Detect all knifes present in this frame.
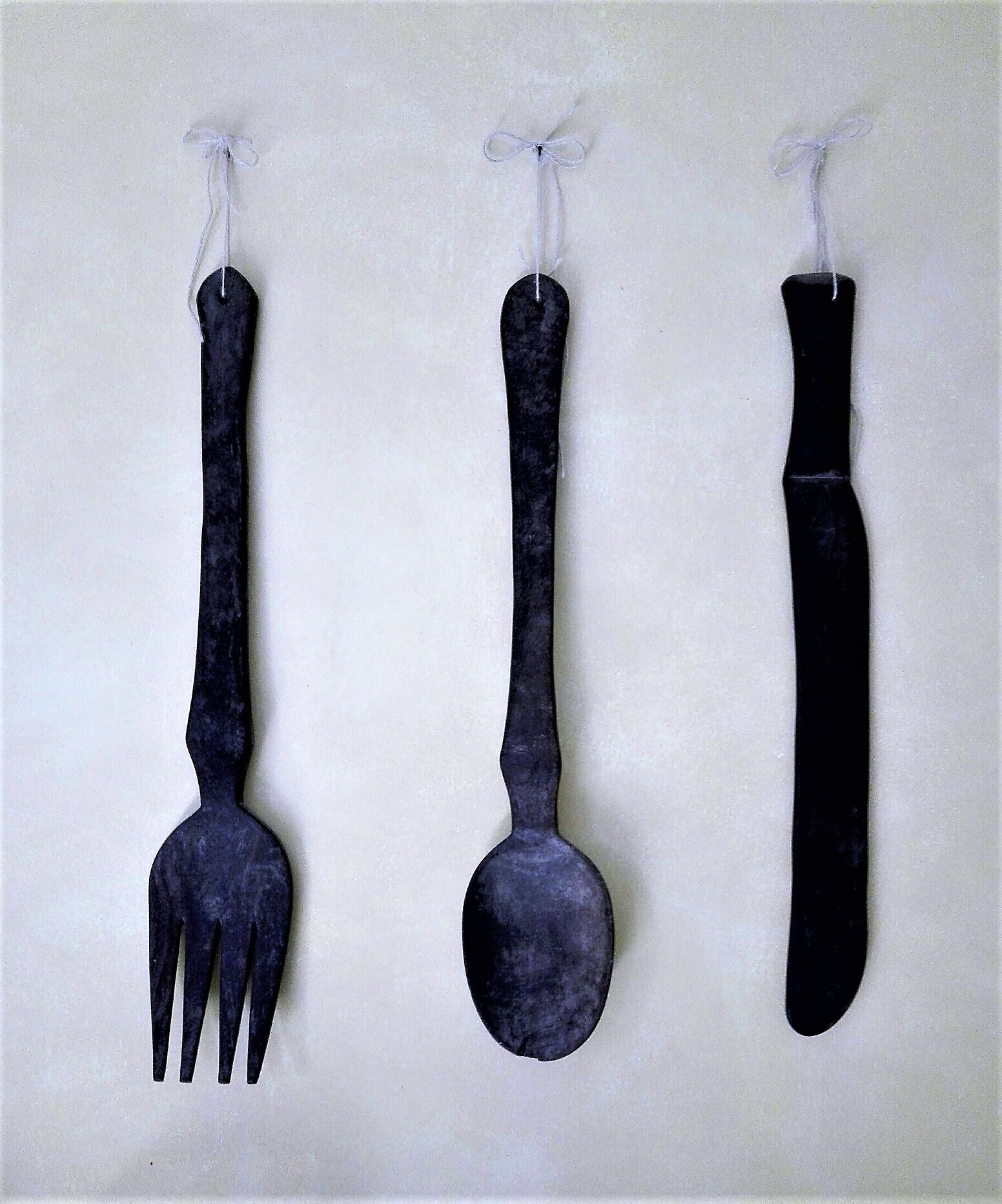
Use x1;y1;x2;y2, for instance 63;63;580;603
780;273;869;1037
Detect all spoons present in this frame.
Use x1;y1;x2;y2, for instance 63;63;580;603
461;271;616;1062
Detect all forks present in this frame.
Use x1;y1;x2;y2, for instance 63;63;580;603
148;265;296;1083
